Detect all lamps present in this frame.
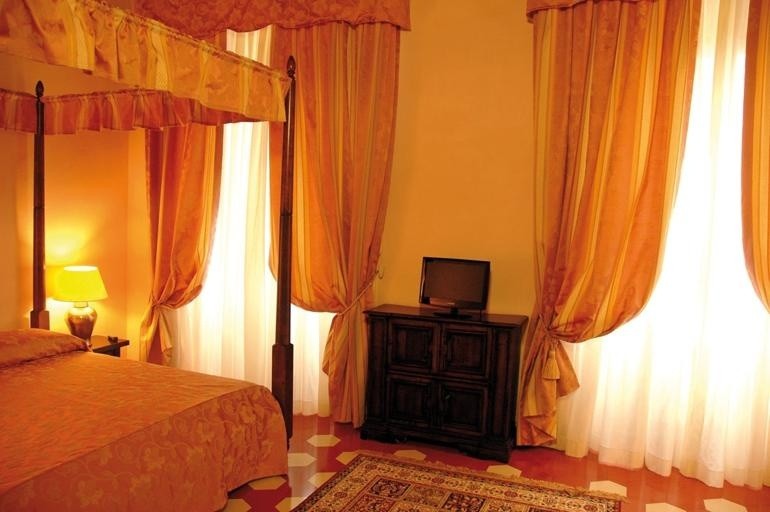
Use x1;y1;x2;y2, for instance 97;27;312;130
51;264;109;337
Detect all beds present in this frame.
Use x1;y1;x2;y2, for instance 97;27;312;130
0;311;295;512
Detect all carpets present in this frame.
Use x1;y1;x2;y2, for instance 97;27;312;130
288;451;623;512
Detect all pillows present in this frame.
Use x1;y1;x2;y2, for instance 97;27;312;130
0;327;91;369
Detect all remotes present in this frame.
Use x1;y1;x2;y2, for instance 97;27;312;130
108;336;118;342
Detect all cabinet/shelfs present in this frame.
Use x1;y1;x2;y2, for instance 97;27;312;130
361;304;529;464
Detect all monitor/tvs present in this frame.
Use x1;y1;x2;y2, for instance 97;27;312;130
419;257;490;319
